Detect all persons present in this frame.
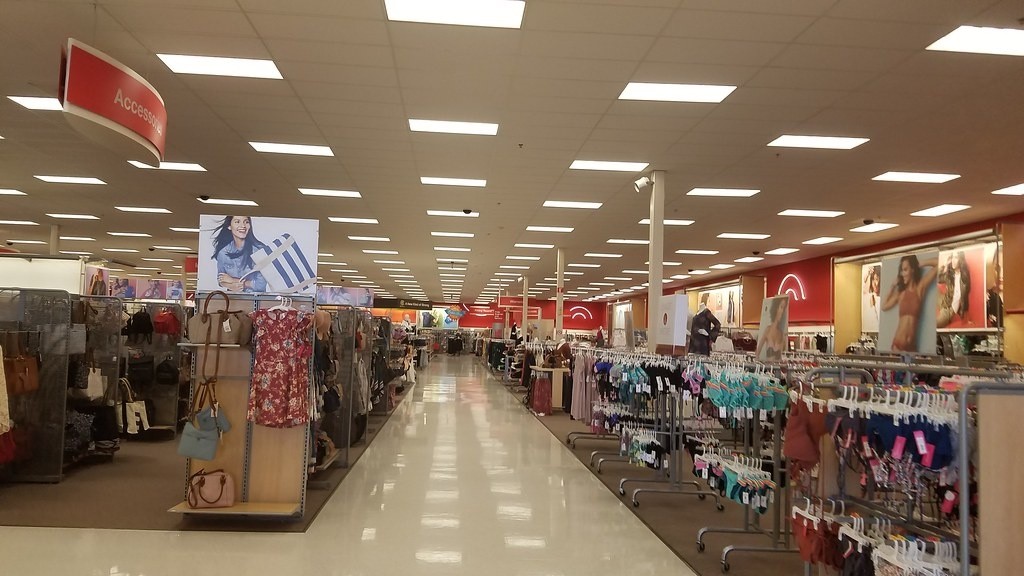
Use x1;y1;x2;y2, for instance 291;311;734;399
596;326;606;344
87;268;106;295
211;217;271;293
688;302;721;354
166;280;183;300
756;299;788;363
863;266;882;326
317;286;374;306
510;322;519;340
141;280;163;299
555;338;573;367
402;313;413;331
883;256;937;351
114;279;134;298
938;253;977;327
987;245;1006;327
726;291;738;326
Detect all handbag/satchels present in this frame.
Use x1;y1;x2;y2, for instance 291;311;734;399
67;348;107;401
38;300;86;356
116;378;150;434
4;330;39;396
187;468;234;509
196;378;232;437
188;291;252;345
83;382;118;442
177;382;218;460
238;232;318;294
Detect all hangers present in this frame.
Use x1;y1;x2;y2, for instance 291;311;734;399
570;346;676;374
592;399;661;447
683;352;1024;433
686;430;771;484
280;297;293;311
266;295;288;312
524;341;557;378
792;497;961;576
286;298;296;311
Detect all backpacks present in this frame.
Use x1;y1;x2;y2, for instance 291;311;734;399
157;357;179;385
132;306;154;345
154;306;181;339
121;305;132;341
128;351;155;386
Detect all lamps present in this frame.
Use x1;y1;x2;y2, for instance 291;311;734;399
633;176;654;193
